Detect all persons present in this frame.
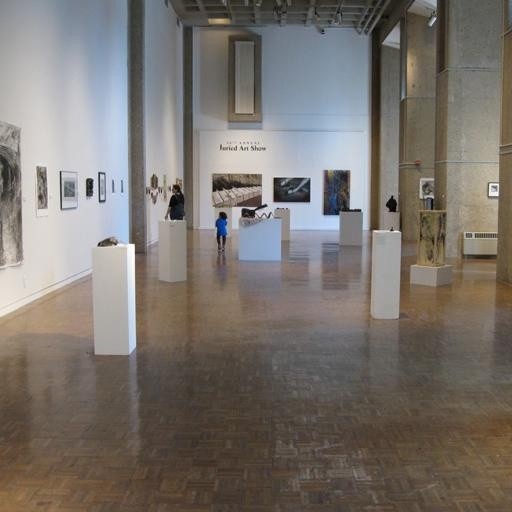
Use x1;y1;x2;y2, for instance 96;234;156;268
164;184;185;220
214;212;228;252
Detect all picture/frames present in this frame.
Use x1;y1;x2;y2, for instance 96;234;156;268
60;170;79;210
419;177;434;200
98;171;107;202
487;182;500;197
